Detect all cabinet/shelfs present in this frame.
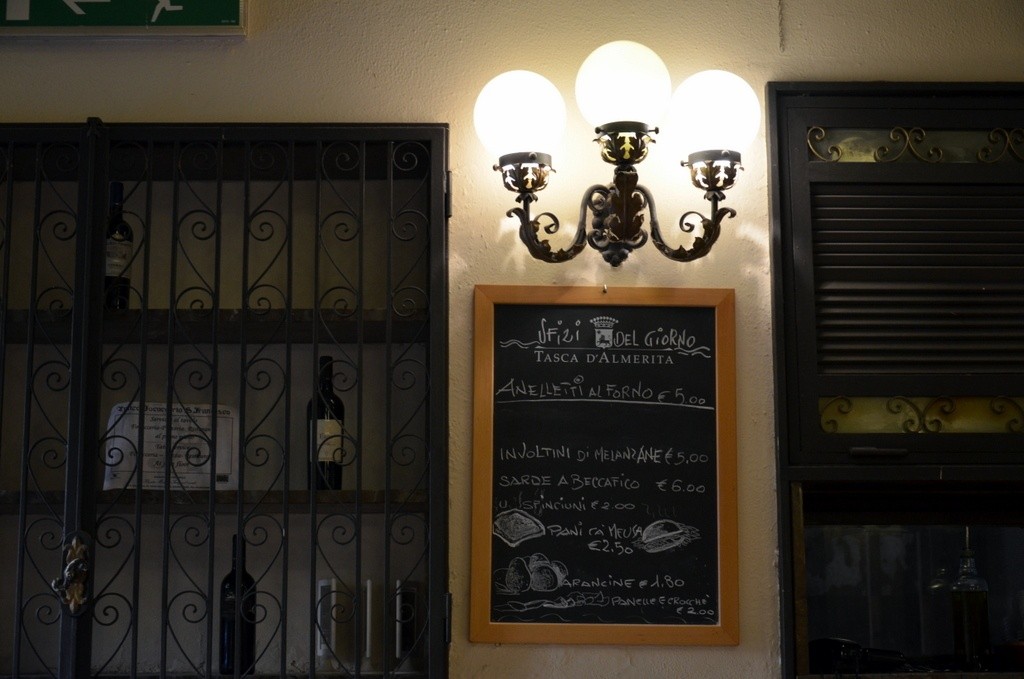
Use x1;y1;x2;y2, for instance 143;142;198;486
2;121;445;679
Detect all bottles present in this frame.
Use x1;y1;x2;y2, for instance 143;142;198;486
306;355;344;491
103;181;134;311
219;533;257;676
951;526;994;672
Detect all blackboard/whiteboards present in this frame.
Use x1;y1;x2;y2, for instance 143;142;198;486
469;284;741;647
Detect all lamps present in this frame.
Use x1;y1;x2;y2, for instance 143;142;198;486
472;40;760;268
